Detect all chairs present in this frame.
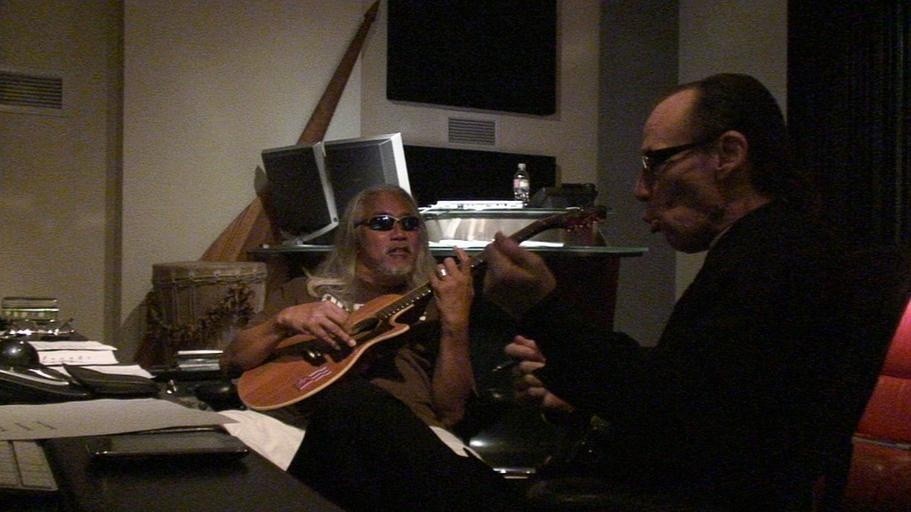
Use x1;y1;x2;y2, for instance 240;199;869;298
812;259;911;511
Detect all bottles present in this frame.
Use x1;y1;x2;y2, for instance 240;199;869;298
514;163;529;207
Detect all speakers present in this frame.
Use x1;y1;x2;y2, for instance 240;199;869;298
260;140;339;245
323;131;412;222
403;144;557;207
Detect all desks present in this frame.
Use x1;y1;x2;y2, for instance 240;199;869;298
0;396;345;512
246;244;649;330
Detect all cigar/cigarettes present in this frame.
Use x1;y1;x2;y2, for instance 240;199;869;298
492;356;520;374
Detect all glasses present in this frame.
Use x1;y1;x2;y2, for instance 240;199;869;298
640;137;708;171
353;213;420;233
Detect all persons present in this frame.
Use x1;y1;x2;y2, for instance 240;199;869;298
216;183;479;512
481;72;892;511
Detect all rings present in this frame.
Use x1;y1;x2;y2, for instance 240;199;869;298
439;269;450;278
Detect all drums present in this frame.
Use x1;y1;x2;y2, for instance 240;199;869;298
153;262;267;366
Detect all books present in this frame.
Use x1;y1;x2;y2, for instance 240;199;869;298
435;198;524;211
1;295;224;396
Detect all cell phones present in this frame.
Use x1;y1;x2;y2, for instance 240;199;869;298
82;432;253;465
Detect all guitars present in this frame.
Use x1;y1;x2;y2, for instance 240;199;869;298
238;206;612;424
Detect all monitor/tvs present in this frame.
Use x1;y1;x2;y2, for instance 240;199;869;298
386;0;558;116
